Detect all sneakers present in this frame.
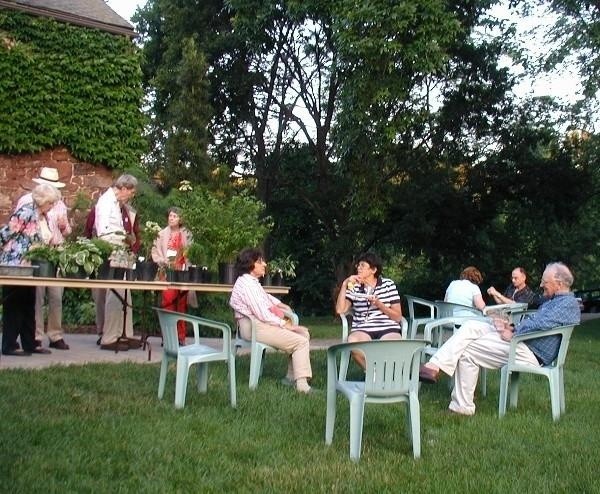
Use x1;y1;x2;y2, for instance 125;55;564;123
3;331;143;356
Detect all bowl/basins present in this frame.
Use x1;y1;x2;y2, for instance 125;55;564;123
0;264;40;277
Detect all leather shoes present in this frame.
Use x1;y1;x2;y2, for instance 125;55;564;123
417;365;440;382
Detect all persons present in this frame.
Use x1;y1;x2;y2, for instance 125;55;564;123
419;260;582;416
336;251;403;371
149;206;196;347
0;181;62;356
93;172;141;351
486;266;536;327
229;246;323;394
14;167;72;349
85;179;140;345
443;265;487;330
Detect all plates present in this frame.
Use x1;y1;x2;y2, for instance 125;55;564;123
350;292;375;298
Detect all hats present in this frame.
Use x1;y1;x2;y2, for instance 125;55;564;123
31;168;66;188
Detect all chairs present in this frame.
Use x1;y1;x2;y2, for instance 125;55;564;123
151;307;237;409
233;306;300;390
404;294;440;342
422;317;493;398
487;303;529;328
337;310;409;385
324;338;431;460
497;322;582;422
435;299;484;347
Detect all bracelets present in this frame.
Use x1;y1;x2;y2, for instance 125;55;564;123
497;293;503;298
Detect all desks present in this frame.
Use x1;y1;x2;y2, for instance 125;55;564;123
1;275;292;360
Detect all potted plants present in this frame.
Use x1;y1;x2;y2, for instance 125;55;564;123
27;179;298;286
